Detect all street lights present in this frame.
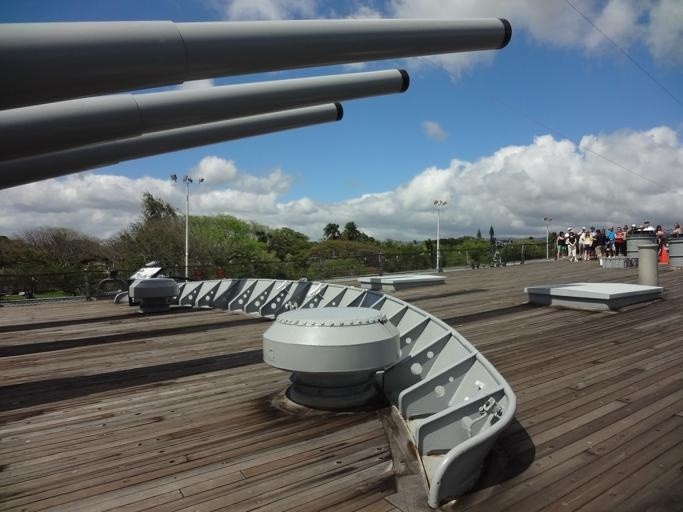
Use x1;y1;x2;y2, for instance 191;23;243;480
544;217;553;260
434;199;448;268
170;174;204;283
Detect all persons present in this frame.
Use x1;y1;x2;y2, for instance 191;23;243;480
556;222;683;266
